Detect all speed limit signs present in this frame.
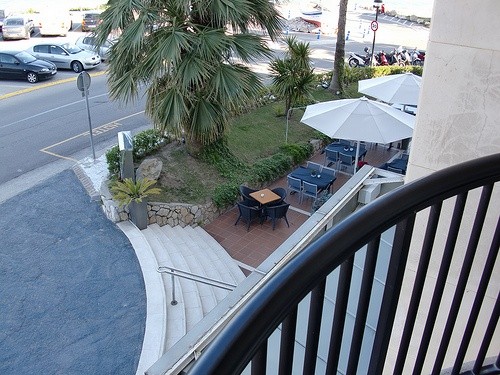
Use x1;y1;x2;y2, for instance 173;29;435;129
371;20;378;31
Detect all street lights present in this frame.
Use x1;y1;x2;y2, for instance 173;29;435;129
370;0;382;69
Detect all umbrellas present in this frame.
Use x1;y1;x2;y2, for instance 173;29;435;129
299;96;415;173
359;72;422;112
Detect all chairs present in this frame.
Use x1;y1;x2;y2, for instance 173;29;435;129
400;154;409;162
388;167;402;174
234;139;368;232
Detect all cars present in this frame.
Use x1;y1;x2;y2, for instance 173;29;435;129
38;10;74;36
0;50;58;84
1;15;35;40
23;41;102;73
0;9;8;31
81;12;103;32
74;33;119;61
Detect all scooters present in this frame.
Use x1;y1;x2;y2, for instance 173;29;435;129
347;47;426;68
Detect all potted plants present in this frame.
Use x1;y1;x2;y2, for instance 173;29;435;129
112;176;163;231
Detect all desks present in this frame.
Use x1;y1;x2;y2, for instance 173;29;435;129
326;143;368;170
292;168;336;202
390;159;407;171
249;188;282;225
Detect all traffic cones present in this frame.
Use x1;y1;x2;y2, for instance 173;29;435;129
346;31;351;40
316;30;321;39
285;27;289;35
